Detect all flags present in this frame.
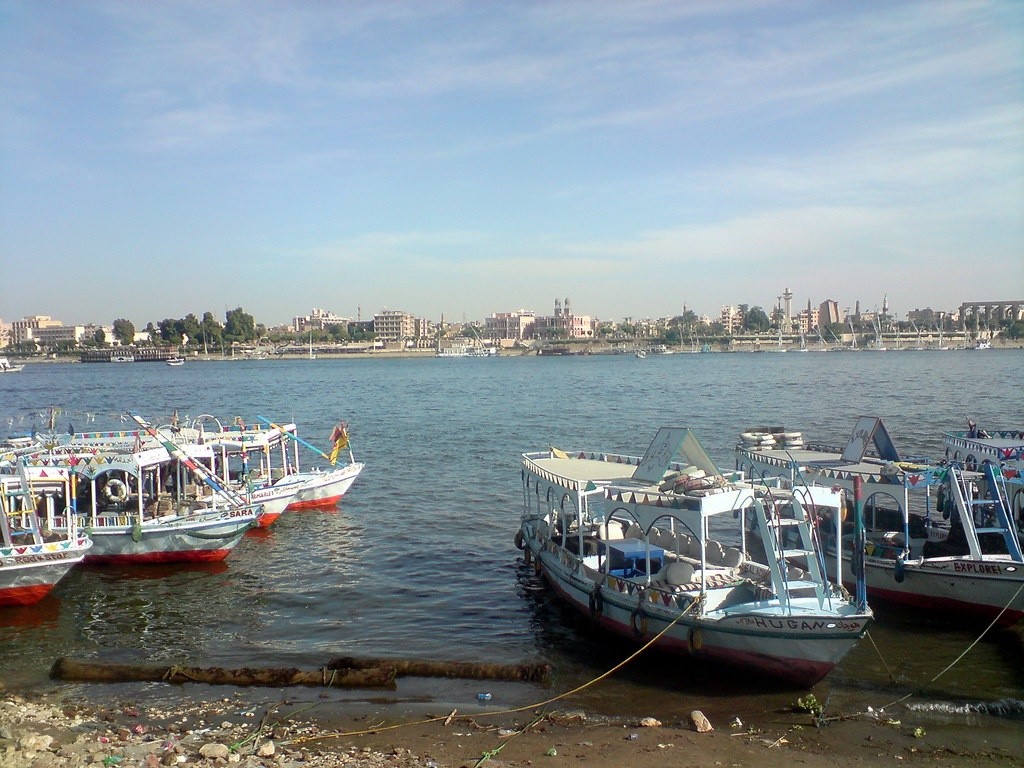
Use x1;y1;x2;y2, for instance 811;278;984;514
329;423;350;466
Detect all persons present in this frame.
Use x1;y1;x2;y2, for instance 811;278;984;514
763;499;855;537
38;456;220;519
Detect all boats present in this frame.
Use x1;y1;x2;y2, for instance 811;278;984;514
942;415;1024;544
111;355;136;363
736;414;1024;625
0;355;26;373
165;358;184;365
634;350;646;359
0;410;365;609
434;345;490;357
512;428;874;690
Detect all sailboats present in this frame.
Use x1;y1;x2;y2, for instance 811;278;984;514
767;317;991;352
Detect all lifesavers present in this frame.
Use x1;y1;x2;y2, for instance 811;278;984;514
741;430;805;452
683;625;701;656
105;479;127;503
533;555;543;579
523;544;532;566
629;606;648;637
659;465;736;498
514;532;527;551
587;589;603;620
